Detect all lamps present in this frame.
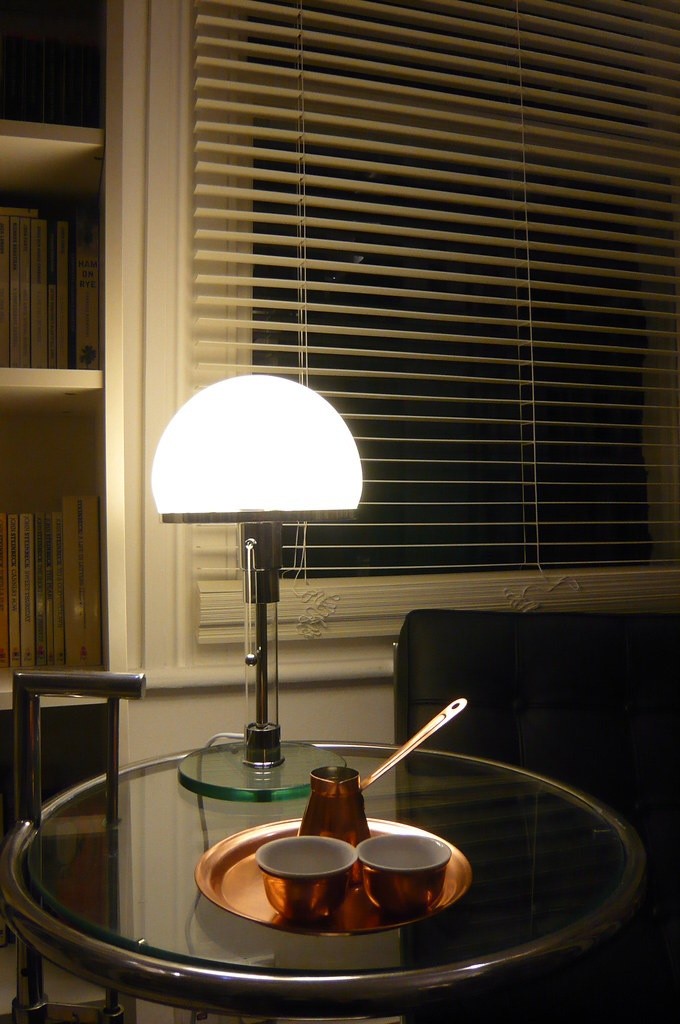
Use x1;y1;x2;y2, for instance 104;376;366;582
151;375;364;800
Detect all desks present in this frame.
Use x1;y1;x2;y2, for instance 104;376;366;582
0;741;647;1024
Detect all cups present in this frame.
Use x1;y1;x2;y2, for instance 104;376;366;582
358;835;452;913
258;837;357;924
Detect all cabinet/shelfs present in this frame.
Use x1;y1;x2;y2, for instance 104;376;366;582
0;0;124;953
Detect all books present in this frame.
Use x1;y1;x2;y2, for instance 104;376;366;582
0;206;105;370
0;495;101;668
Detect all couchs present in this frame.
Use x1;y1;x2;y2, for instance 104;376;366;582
395;608;680;1024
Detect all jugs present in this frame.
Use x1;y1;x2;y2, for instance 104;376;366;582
297;698;467;888
